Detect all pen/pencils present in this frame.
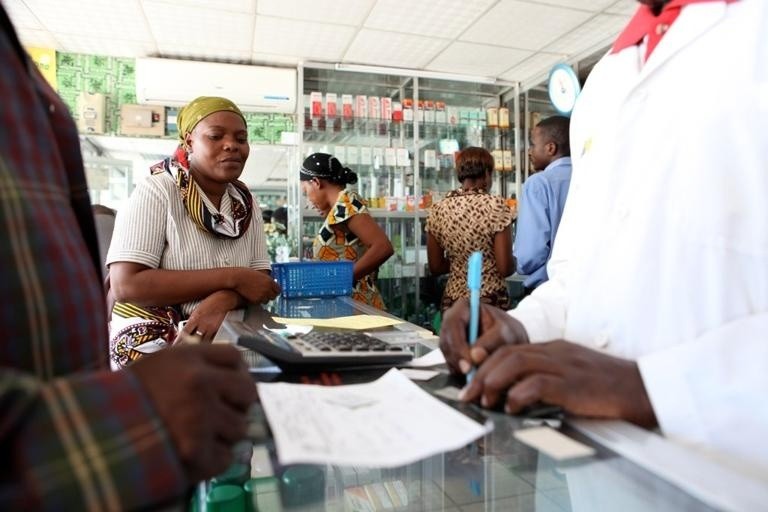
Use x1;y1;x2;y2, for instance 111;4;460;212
467;251;483;386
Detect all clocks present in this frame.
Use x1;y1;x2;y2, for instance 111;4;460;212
545;63;579;119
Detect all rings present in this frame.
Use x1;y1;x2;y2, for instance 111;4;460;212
194;328;205;338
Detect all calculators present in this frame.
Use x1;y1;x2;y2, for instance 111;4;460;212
223;322;415;368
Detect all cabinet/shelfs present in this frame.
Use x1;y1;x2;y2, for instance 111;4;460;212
301;69;513;333
157;292;767;512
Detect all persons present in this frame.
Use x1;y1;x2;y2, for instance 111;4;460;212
298;152;396;313
436;1;768;435
426;143;517;325
105;93;283;374
512;114;575;298
0;3;263;512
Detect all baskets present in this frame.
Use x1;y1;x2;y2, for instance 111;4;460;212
270;261;352;298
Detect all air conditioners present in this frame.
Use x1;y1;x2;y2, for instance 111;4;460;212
133;55;296;114
